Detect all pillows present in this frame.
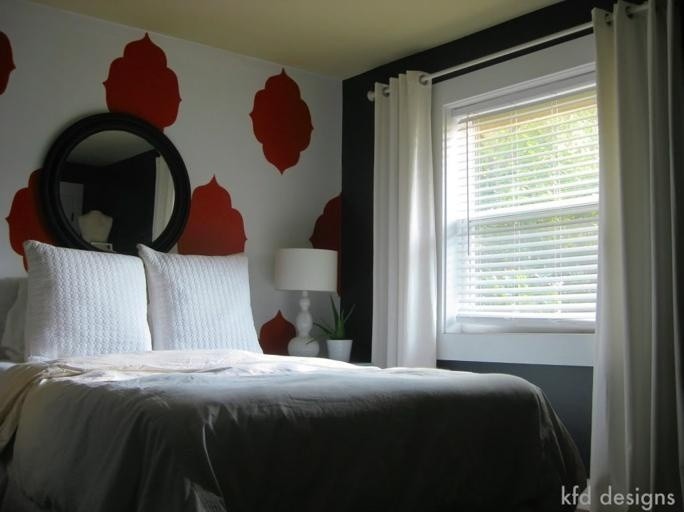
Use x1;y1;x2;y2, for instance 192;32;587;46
22;239;263;362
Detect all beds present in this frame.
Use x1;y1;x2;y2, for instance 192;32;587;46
0;352;549;512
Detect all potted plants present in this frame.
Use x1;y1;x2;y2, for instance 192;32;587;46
305;294;356;363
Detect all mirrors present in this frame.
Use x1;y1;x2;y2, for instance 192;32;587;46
40;112;190;258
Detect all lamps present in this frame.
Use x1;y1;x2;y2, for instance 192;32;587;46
273;247;339;356
